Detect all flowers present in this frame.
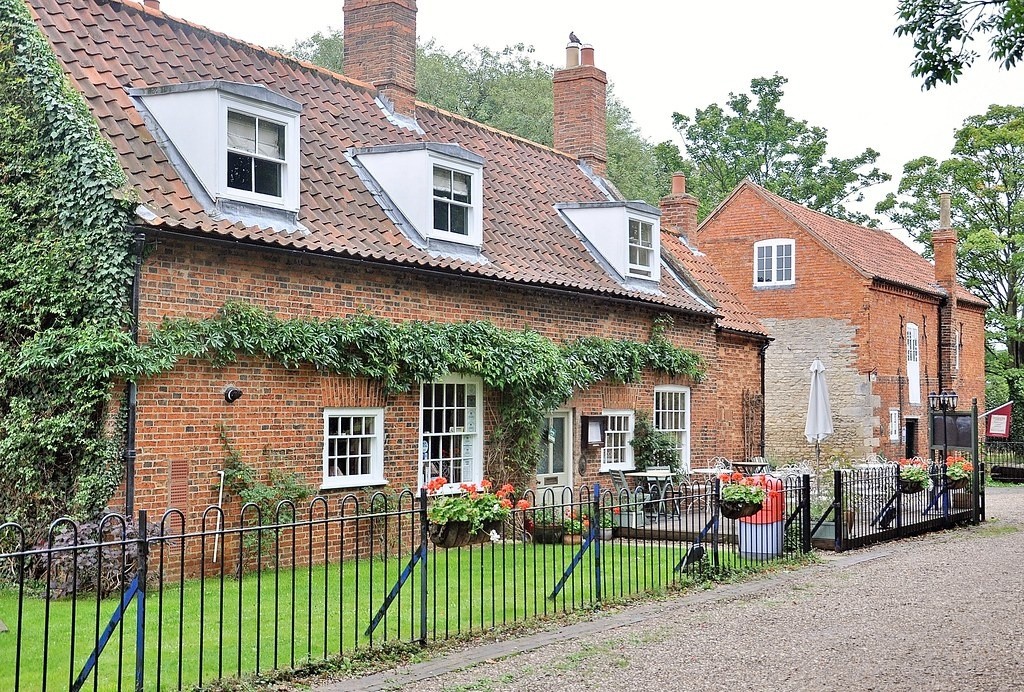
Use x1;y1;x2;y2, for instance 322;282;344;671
945;456;974;481
898;458;931;489
424;477;529;544
720;473;779;505
530;502;621;535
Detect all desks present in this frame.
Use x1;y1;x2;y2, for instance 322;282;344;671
695;469;733;473
625;472;677;518
732;461;769;477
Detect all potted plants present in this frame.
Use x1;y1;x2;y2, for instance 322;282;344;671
609;504;644;529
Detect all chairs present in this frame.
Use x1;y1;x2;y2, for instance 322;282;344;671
680;456;932;515
609;465;681;524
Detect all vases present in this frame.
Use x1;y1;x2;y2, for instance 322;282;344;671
428;520;504;548
947;477;968;489
535;525;562;543
900;478;930;494
719;499;763;518
600;528;613;540
563;534;583;545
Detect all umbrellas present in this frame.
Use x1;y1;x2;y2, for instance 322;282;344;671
804;356;835;500
